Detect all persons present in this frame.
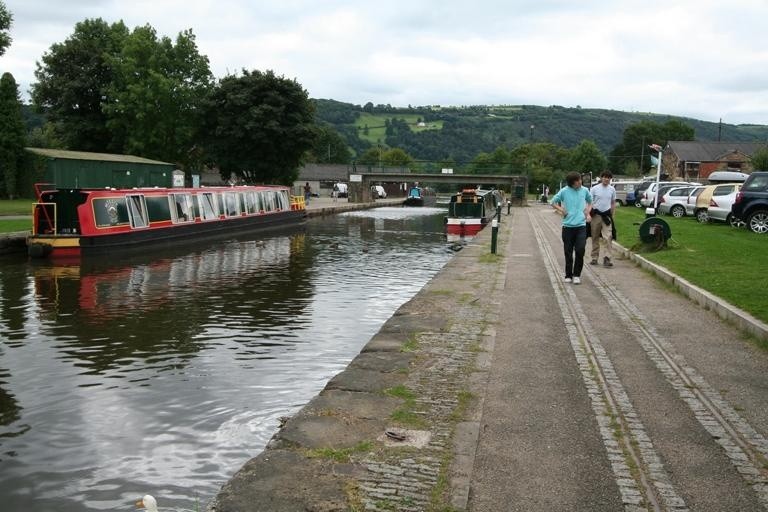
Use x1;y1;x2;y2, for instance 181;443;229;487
589;169;617;266
304;181;310;205
332;185;339;202
548;171;593;283
535;184;540;200
544;186;548;198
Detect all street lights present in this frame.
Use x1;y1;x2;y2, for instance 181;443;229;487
646;141;663;215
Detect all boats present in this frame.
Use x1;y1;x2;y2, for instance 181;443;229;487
29;228;312;320
402;186;438;207
26;183;311;252
444;224;482;254
443;186;507;227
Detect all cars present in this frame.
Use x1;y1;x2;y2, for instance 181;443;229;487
333;183;348;197
370;185;387;199
310;190;321;198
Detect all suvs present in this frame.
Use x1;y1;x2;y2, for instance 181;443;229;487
595;168;749;235
730;171;767;234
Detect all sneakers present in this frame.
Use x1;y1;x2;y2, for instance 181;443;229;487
603;257;613;266
573;277;580;284
590;260;598;265
565;278;572;282
